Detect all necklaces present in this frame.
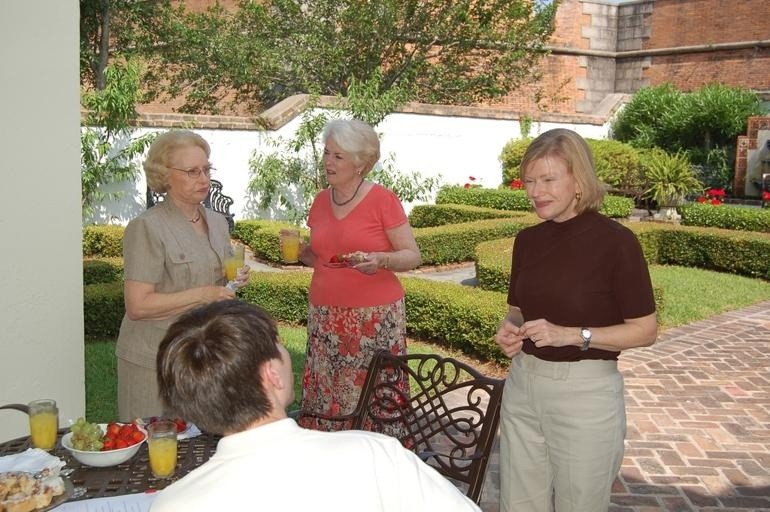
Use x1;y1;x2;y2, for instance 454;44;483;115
329;174;367;207
181;208;206;226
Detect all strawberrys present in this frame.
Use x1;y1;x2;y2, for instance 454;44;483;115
101;424;142;451
328;253;363;267
147;415;183;436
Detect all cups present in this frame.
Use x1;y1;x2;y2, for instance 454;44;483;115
222;245;245;283
27;399;60;451
147;420;177;477
280;229;300;263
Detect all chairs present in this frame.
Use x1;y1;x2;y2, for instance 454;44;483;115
292;347;505;508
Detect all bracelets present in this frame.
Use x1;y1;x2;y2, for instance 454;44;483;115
382;252;390;272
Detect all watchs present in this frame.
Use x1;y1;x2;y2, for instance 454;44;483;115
578;324;594;355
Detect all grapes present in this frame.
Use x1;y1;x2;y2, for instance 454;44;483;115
73;417;103;453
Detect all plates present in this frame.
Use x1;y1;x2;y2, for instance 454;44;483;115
146;415;192;434
0;473;76;512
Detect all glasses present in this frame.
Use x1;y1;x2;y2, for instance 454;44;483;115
166;166;218;179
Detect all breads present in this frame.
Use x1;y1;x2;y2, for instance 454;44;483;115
0;472;64;512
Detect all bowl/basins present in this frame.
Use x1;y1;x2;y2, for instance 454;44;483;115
59;422;149;468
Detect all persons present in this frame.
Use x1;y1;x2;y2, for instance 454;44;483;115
291;112;425;456
138;297;490;512
496;126;663;511
108;125;256;430
